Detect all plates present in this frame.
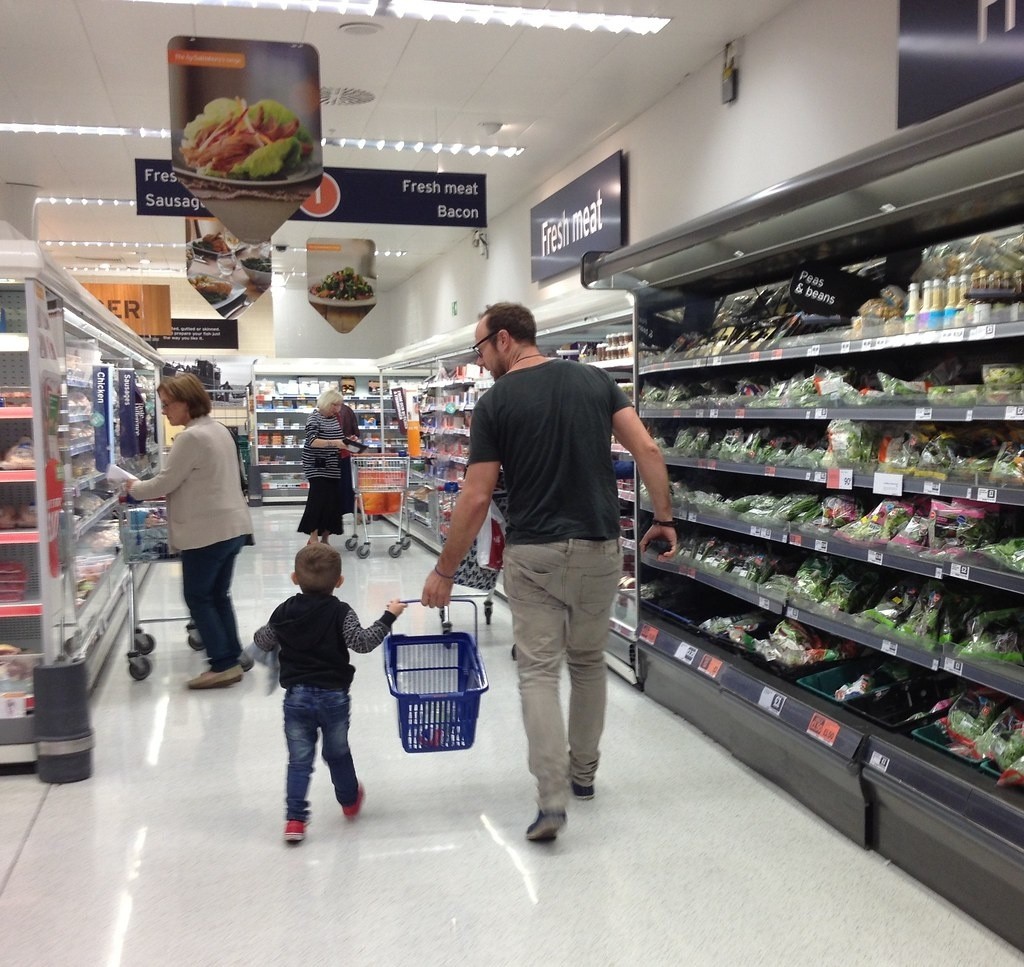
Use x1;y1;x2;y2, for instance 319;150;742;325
307;290;377;307
186;273;247;316
187;238;247;256
171;128;322;186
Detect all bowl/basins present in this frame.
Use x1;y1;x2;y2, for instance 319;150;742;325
241;263;272;284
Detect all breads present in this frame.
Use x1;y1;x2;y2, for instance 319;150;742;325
0;437;37;527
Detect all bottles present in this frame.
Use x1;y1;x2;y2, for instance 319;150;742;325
904;270;1024;334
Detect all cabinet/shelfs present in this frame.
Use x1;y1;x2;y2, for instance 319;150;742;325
0;81;1024;967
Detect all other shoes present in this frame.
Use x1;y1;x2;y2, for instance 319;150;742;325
201;654;255;676
342;782;365;819
570;781;595;800
525;810;566;840
284;821;306;841
188;664;243;688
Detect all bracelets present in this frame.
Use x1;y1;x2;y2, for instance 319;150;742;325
651;518;678;527
435;566;455;578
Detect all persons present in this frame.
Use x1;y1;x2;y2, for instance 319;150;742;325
254;543;408;841
422;303;677;841
297;390;358;546
126;374;256;689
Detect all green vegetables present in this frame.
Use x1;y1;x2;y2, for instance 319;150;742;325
317;267;374;300
181;96;310;180
244;257;271;273
643;367;1024;786
192;241;213;248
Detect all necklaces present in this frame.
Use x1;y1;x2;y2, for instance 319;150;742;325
516;355;545;362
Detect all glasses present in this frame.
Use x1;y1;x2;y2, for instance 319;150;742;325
472;329;500;358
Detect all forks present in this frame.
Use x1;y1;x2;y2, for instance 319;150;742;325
223;294;255;318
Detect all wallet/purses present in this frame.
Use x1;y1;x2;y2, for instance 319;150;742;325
314;457;326;468
